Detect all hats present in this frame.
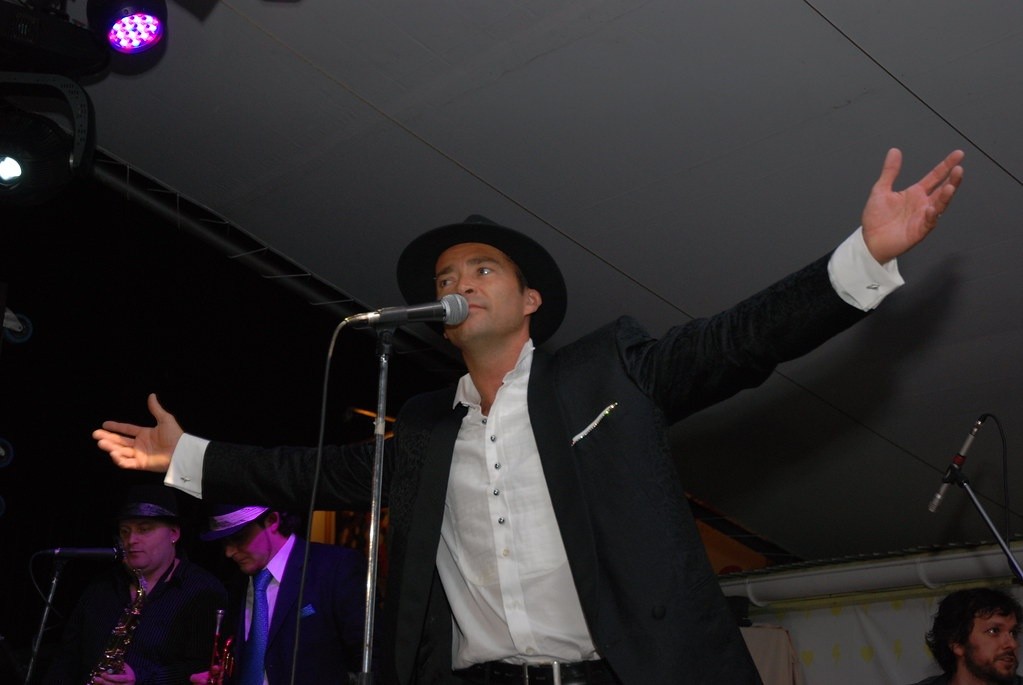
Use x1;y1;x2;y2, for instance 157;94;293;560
396;214;568;346
200;491;271;541
112;486;181;523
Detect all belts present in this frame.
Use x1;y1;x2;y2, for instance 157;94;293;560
452;658;617;685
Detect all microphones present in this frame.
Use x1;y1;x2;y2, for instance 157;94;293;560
41;548;118;559
345;293;469;326
928;413;989;513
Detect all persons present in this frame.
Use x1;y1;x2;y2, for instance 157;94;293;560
93;147;965;685
42;487;384;685
903;581;1023;685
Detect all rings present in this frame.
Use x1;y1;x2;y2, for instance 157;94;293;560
937;212;943;220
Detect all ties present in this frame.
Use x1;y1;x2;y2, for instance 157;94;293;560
239;568;273;685
